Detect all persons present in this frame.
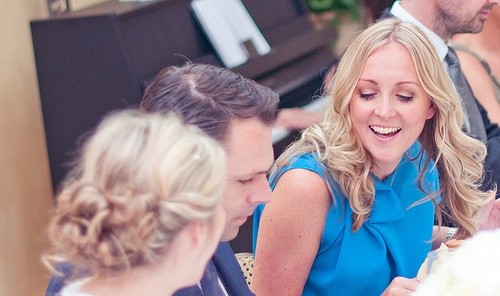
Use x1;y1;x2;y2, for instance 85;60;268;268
251;18;500;296
381;0;500;227
41;108;228;296
45;64;280;296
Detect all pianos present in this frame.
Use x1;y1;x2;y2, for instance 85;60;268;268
28;0;339;254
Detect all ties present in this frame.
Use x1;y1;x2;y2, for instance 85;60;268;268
200;257;226;296
444;51;488;145
